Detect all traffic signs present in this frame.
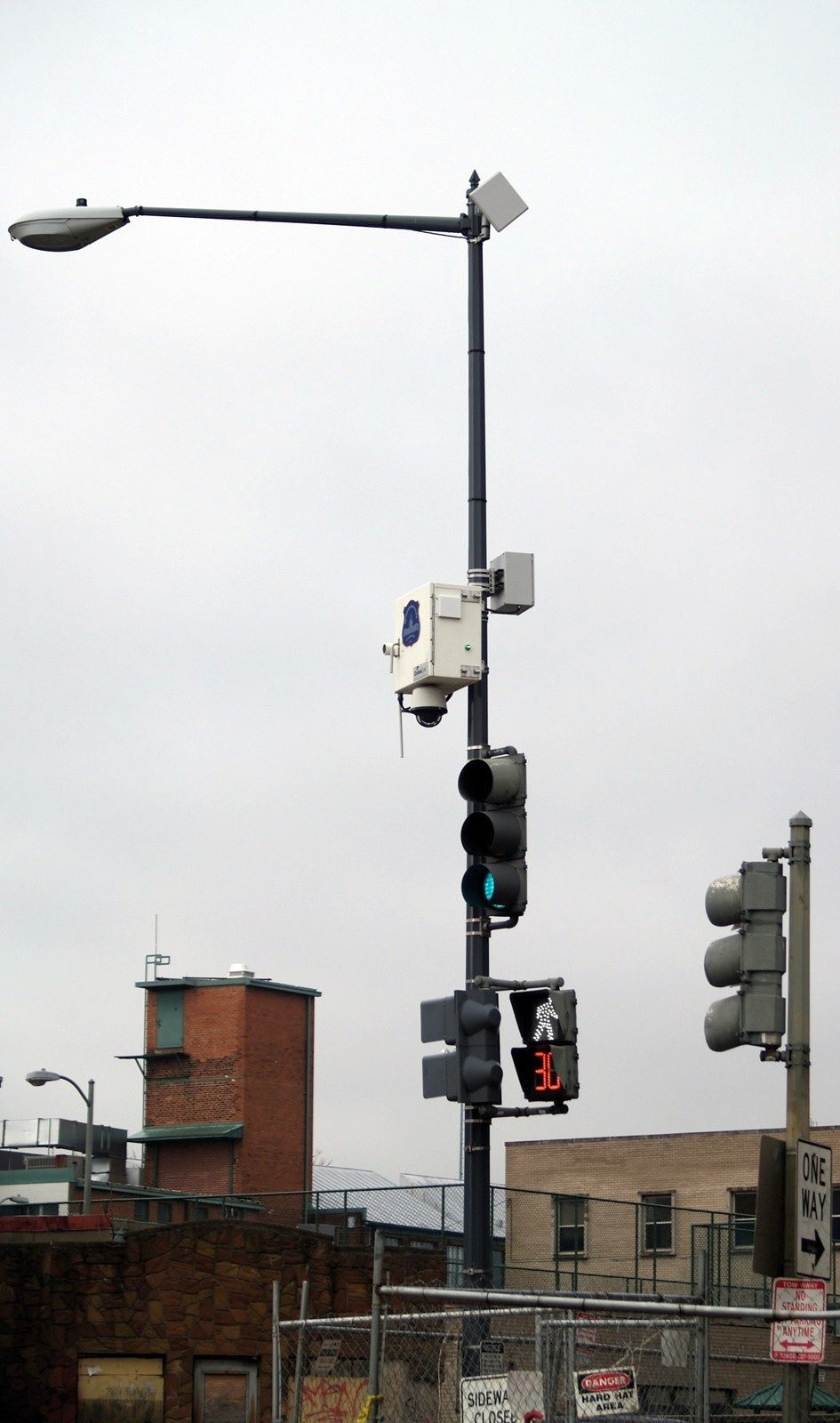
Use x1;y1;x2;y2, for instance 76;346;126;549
794;1136;834;1282
768;1277;827;1364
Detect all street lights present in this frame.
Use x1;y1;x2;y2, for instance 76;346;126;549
7;166;541;1377
25;1070;95;1215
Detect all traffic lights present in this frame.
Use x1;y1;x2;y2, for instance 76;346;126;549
456;751;528;917
419;987;501;1109
507;989;579;1102
702;860;788;1054
